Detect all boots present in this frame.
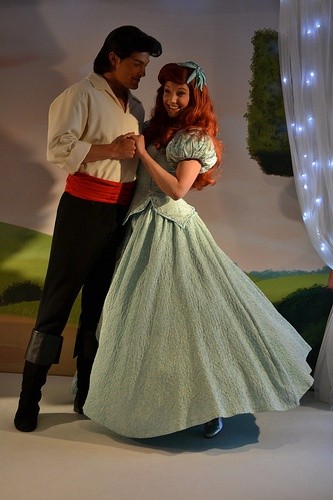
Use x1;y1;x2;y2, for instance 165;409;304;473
74;333;98;413
14;329;64;430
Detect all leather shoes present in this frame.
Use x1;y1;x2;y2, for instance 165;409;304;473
205;417;223;439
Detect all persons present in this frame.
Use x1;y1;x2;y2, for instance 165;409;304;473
81;60;315;440
12;24;162;432
